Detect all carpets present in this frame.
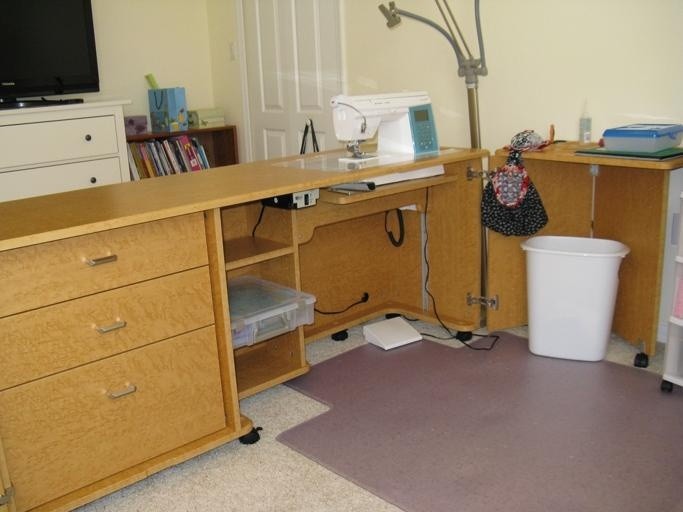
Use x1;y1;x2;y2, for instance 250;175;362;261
274;328;682;512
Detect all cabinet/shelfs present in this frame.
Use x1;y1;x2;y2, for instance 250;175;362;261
660;191;683;388
125;124;239;175
0;99;134;204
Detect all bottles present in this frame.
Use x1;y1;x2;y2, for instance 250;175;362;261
578;101;592;147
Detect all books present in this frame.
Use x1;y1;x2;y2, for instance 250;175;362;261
125;135;211;182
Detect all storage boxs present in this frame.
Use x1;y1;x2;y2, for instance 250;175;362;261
600;124;683;154
226;274;317;350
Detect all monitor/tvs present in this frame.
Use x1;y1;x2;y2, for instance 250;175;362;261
0;0;99;110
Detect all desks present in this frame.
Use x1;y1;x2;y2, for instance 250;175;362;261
0;144;490;512
483;140;683;367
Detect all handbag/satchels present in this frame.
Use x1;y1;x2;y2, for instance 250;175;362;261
481;158;547;236
148;88;188;133
